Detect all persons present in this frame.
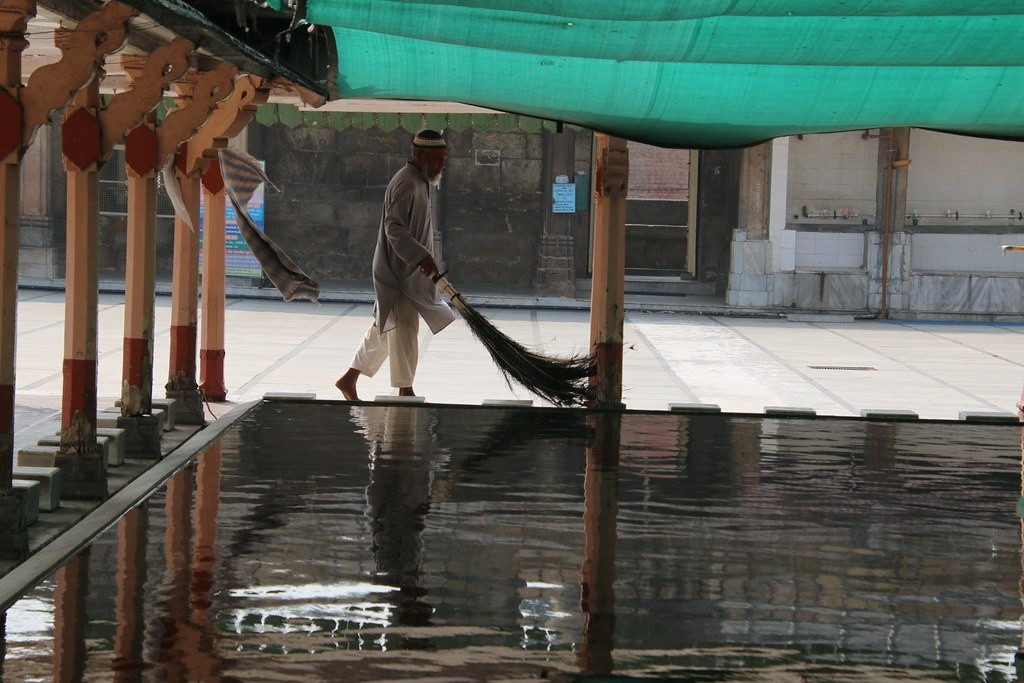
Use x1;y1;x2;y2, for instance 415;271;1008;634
335;130;455;401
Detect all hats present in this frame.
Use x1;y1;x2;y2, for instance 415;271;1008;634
411;130;448;149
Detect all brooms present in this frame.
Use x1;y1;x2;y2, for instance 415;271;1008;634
417;261;597;412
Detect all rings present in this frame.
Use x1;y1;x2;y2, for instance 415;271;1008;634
422;268;425;271
